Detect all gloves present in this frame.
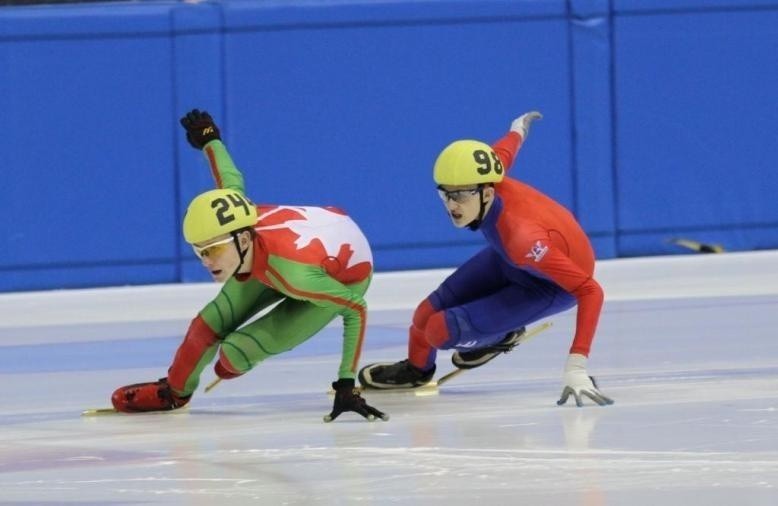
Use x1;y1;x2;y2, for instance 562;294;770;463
319;376;391;422
556;352;615;408
509;109;544;139
179;107;221;150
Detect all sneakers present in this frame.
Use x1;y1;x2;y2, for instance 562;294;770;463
450;327;525;371
111;378;193;413
212;359;245;379
358;357;437;391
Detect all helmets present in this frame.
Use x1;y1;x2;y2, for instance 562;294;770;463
181;186;258;245
433;138;506;188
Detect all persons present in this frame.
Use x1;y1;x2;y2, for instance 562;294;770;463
112;110;391;419
356;111;617;407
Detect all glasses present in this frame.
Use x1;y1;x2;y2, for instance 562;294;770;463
435;185;481;201
192;235;245;258
